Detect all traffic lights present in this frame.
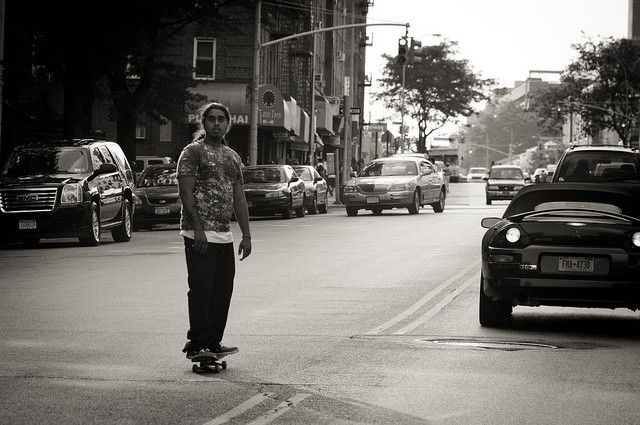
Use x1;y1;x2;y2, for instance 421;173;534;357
398;37;408;64
410;39;422;67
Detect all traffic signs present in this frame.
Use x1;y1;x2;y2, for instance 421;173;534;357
339;107;362;114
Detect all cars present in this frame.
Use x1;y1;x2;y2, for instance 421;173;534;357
131;164;192;232
482;164;529;204
478;182;639;325
293;166;328;214
232;165;307;220
340;153;447;215
530;164;556;182
467;167;489;183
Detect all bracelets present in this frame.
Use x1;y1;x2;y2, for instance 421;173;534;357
242;235;251;240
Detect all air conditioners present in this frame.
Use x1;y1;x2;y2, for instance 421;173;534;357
315;74;322;81
341;8;347;18
339;52;345;62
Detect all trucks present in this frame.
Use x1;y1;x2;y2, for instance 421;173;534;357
427;133;460;183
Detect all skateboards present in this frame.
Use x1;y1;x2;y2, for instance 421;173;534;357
191;352;228;373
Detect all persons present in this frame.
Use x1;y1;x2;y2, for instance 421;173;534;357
352;159;364;173
177;102;252;358
316;157;335;196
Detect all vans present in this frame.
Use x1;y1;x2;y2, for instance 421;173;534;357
551;145;639;182
129;156;172;176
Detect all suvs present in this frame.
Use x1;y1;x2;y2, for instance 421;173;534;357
0;138;135;245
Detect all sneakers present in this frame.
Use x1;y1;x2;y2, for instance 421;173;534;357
186;348;218;358
212;346;239;354
